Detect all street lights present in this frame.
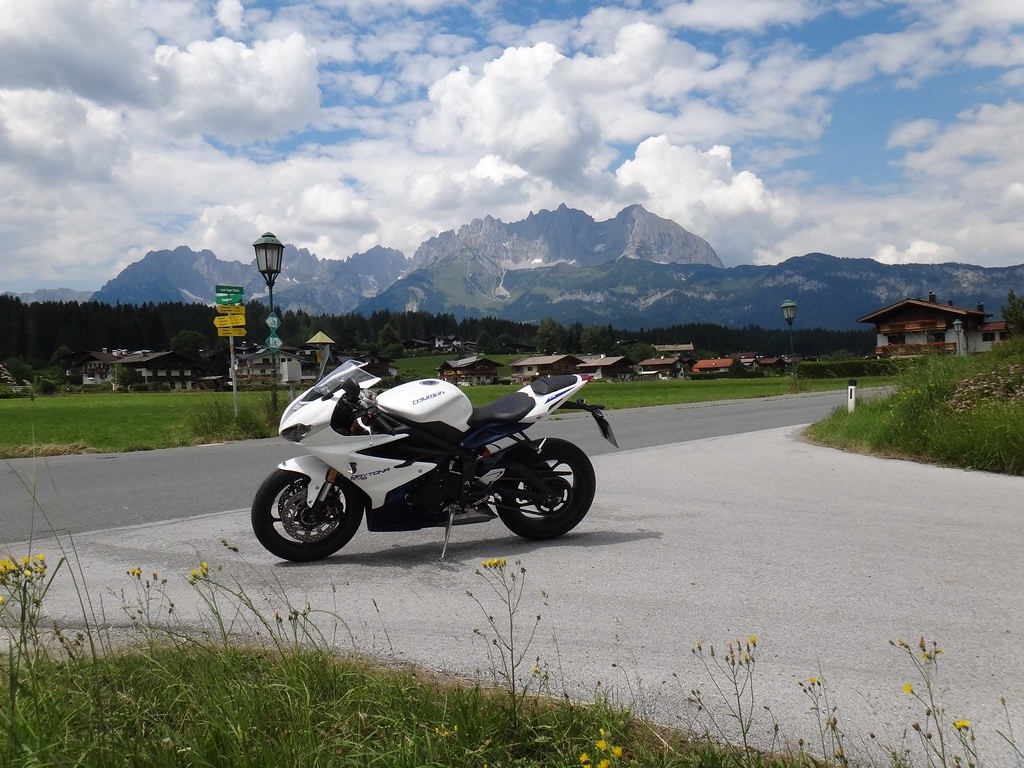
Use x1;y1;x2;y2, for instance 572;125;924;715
953;319;962;355
252;229;286;409
779;299;800;394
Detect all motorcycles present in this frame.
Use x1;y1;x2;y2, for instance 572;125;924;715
249;359;620;562
